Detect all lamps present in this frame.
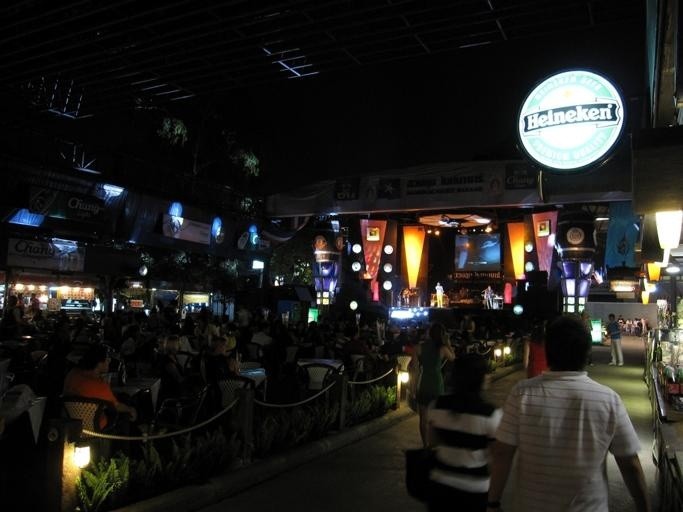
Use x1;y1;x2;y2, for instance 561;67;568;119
165;215;184;234
641;290;649;305
647;262;661;284
69;420;94;470
515;70;633;180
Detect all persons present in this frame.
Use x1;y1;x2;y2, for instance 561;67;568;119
528;320;547;377
403;287;415;307
435;282;445;308
0;291;63;348
606;313;624;366
582;310;596;367
415;321;454;448
138;299;237;377
63;311;138;343
426;353;503;512
63;344;138;431
237;304;414;374
487;316;651;512
615;309;665;338
485;286;495;309
455;311;486;354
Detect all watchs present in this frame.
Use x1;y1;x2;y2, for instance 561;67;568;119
487;500;501;508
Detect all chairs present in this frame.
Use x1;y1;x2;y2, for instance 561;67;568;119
397;354;413;372
59;393;129;458
216;374;257;411
302;362;337;407
